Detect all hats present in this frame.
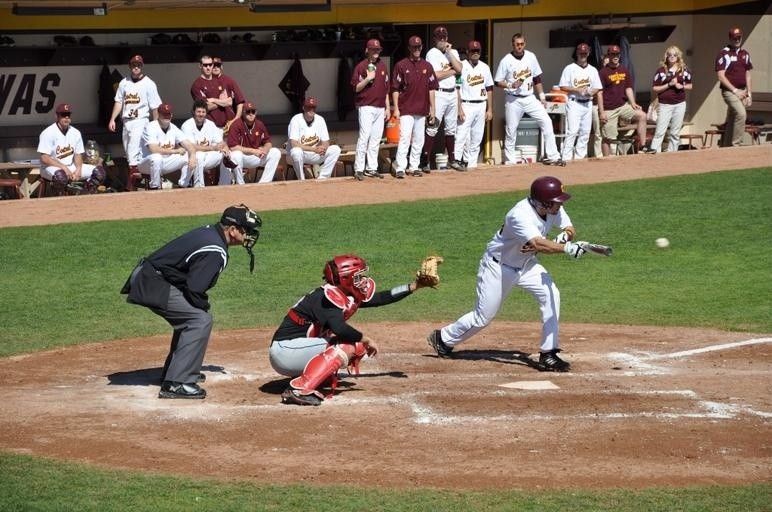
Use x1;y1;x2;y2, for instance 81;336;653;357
367;39;382;49
576;43;591;54
130;55;144;67
242;102;258;110
304;95;318;108
223;156;238;169
408;26;481;51
56;103;72;113
729;26;743;41
607;45;622;54
158;103;175;113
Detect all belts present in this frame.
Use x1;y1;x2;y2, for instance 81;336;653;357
462;100;487;103
572;97;590;103
436;87;454;92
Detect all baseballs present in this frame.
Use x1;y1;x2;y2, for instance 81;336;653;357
657;237;669;248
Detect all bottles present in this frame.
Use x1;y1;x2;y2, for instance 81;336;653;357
225;27;232;44
367;60;375;84
85;139;100;165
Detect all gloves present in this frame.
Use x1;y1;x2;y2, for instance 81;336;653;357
552;231;571;244
563;241;589;257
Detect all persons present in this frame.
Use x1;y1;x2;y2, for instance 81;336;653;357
108;54;281;190
286;96;342;181
454;41;494;169
423;28;463;173
650;46;692;153
351;40;390;180
494;32;563;165
36;103;107;195
426;175;586;371
119;203;261;398
559;44;603;160
713;26;754;147
391;35;439;179
585;46;656;159
269;253;423;406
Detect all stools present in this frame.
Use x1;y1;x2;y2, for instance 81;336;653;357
2;121;760;200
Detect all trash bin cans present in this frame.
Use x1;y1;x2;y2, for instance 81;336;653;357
503;117;540;145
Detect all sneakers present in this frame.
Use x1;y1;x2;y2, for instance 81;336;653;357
637;145;656;153
158;374;206;383
427;329;455;359
159;381;206;399
447;160;468;171
354;165;430;181
537;347;570;372
280;385;321;405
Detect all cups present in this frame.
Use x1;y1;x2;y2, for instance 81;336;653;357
103;151;110;164
335;32;342;40
145;39;152;46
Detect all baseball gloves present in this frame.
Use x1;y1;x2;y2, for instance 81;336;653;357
416;255;444;289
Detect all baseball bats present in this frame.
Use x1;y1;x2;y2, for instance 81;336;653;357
522;244;612;257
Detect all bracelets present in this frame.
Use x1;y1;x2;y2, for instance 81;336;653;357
747;93;752;98
667;83;670;87
733;88;738;93
682;84;685;90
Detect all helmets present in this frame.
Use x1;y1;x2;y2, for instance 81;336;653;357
323;255;369;303
221;204;261;251
530;176;571;203
